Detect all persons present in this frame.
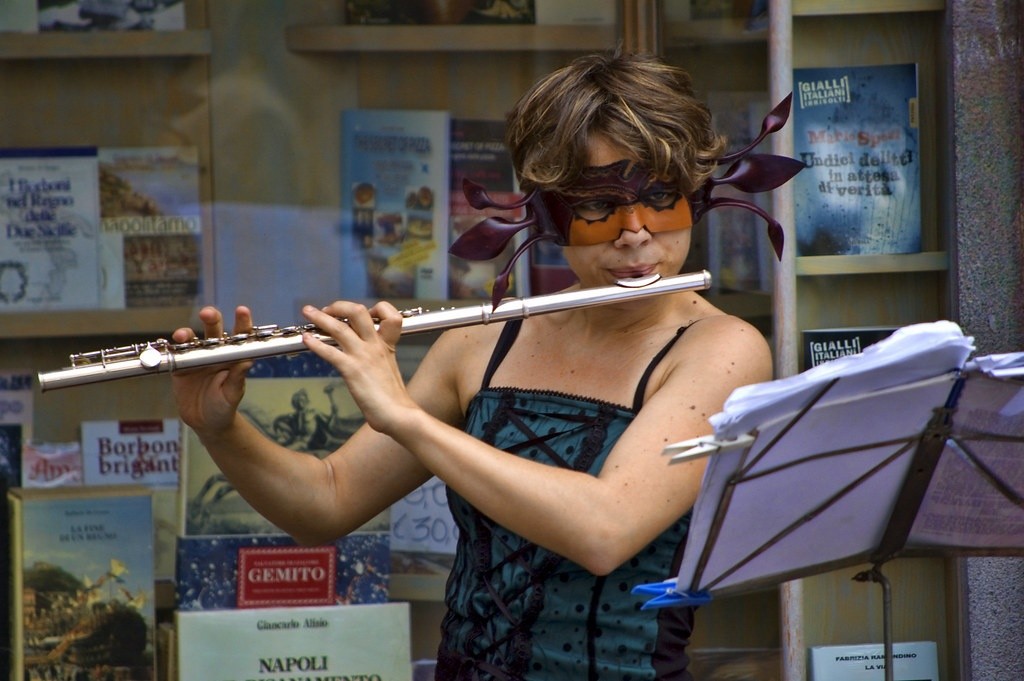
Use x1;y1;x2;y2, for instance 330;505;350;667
169;52;774;681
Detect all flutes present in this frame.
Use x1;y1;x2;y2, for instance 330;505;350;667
35;269;715;403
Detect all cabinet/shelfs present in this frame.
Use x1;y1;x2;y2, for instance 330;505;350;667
0;30;209;336
285;0;958;681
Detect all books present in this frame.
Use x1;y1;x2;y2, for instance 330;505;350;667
0;0;1024;681
